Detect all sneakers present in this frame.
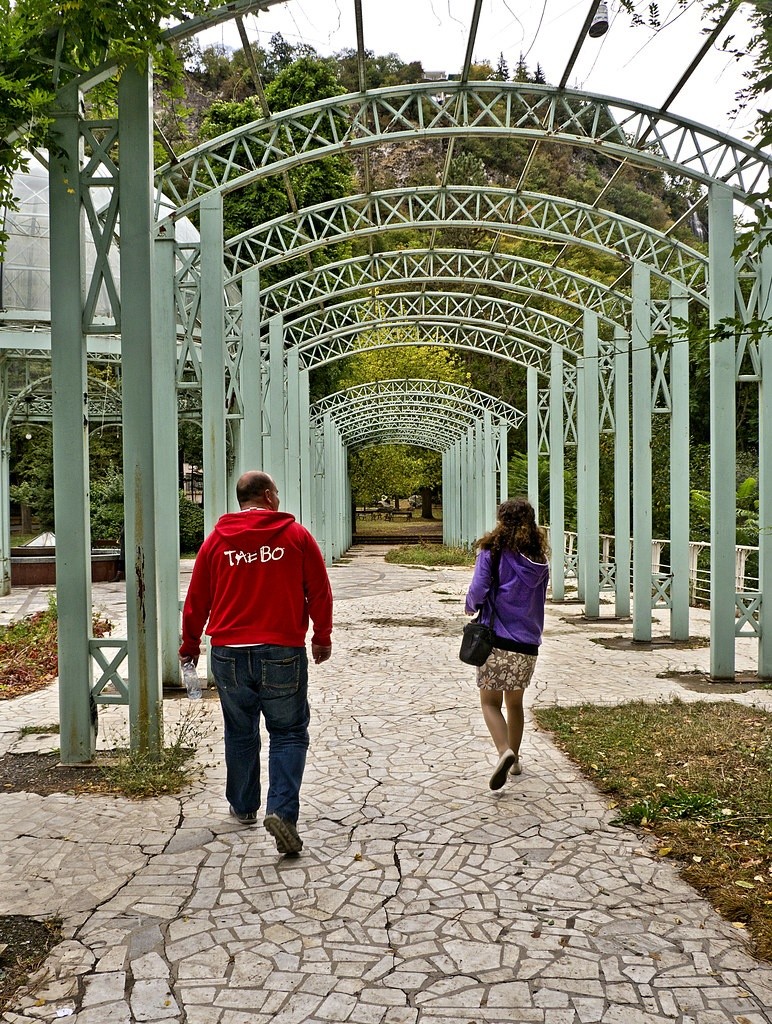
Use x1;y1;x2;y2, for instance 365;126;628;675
264;813;303;853
229;803;258;824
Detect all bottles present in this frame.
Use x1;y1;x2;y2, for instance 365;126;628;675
182;662;203;700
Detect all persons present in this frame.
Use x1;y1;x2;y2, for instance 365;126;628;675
177;470;333;853
107;526;125;582
464;500;550;791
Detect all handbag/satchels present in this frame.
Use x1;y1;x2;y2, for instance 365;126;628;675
459;622;496;667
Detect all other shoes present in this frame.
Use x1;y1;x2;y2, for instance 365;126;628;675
489;749;515;789
511;755;524;775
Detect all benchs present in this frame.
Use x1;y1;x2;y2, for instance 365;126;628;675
356;511;413;522
11;547;124;585
